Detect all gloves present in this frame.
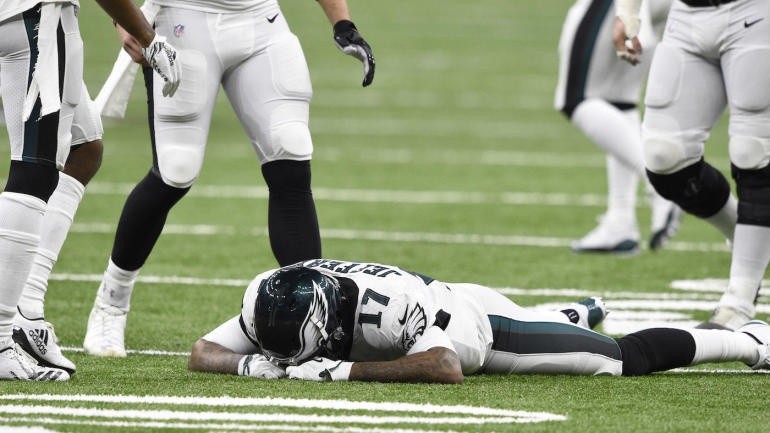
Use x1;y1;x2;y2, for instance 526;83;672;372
285;357;355;381
332;19;375;87
238;353;287;379
143;31;182;97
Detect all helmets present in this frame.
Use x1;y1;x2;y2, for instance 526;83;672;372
252;267;345;370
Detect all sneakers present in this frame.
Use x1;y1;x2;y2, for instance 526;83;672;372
12;305;76;373
83;288;130;357
557;296;610;331
735;319;770;372
0;339;70;381
694;303;755;333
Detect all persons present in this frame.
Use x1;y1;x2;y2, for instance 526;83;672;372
0;0;182;385
189;256;770;384
553;0;684;254
612;0;770;330
83;0;376;358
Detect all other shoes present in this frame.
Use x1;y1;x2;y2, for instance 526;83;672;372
572;220;641;256
650;190;680;252
